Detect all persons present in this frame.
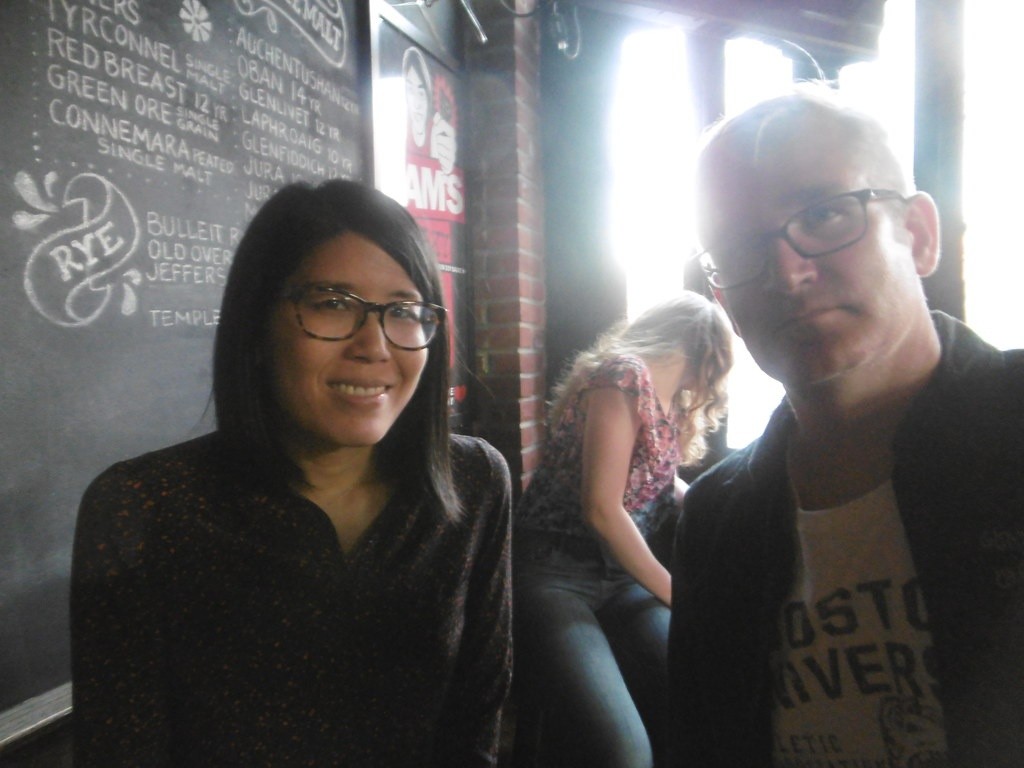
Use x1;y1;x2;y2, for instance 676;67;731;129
67;178;512;768
663;83;1024;768
512;290;735;768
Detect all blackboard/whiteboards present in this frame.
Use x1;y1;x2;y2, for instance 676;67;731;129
0;0;379;747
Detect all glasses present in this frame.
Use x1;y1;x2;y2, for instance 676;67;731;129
268;285;446;351
698;188;906;288
